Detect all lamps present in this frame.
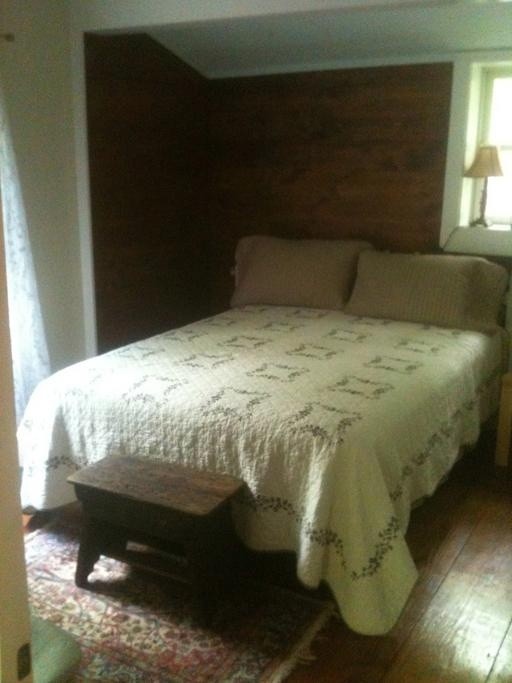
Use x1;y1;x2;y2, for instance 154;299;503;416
461;142;504;228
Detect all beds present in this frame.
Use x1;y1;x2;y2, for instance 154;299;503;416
15;302;512;637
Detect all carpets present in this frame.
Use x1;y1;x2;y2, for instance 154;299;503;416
19;510;334;683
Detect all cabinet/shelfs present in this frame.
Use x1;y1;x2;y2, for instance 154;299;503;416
0;192;34;683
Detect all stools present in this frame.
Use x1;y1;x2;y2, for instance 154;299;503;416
65;454;249;622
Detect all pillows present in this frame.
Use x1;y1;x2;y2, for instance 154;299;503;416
345;244;510;334
227;233;373;311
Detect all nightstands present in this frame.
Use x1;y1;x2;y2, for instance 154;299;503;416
489;371;512;471
